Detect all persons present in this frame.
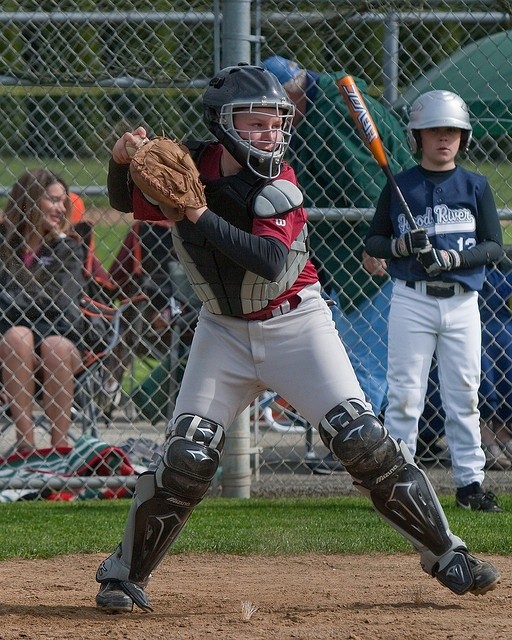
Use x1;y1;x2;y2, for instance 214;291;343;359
94;63;501;617
261;54;419;476
1;170;86;451
362;89;501;512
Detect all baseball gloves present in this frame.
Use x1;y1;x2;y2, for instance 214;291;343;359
130;130;208;222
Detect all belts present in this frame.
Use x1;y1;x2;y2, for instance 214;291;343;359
404;279;469;297
256;297;299;320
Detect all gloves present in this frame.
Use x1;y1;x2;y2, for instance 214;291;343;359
418;247;462;277
391;227;432;259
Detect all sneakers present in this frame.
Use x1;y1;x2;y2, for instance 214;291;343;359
461;547;501;596
458;482;504;513
95;580;133;612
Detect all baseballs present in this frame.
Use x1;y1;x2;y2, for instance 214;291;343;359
124;134;150;159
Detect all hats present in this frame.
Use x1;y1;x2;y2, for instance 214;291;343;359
260;56;300;85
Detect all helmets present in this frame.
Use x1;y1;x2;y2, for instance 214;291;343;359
203;62;295;180
407;90;474;160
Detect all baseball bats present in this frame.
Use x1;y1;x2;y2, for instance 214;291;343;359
337;75;441;278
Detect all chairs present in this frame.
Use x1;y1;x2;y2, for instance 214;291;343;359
1;224;107;439
